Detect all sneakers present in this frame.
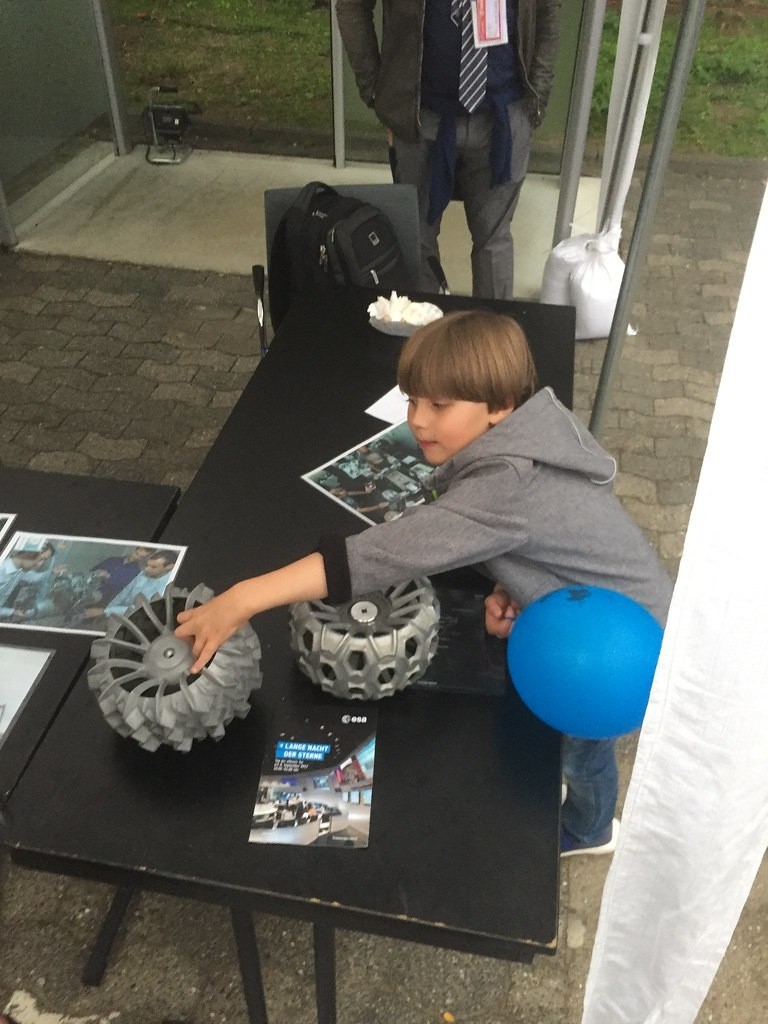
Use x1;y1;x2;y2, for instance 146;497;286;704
559;816;620;858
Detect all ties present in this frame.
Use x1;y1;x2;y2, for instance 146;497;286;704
450;0;488;116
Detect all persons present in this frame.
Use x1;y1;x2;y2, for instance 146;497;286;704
178;310;672;856
0;543;66;617
85;551;178;617
325;445;390;513
336;0;561;294
89;546;157;608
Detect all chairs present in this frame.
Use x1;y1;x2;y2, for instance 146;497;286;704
252;181;452;360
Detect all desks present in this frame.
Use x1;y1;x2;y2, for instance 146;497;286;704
0;282;576;1024
0;468;181;807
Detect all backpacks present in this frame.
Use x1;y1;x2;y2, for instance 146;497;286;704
268;181;411;336
